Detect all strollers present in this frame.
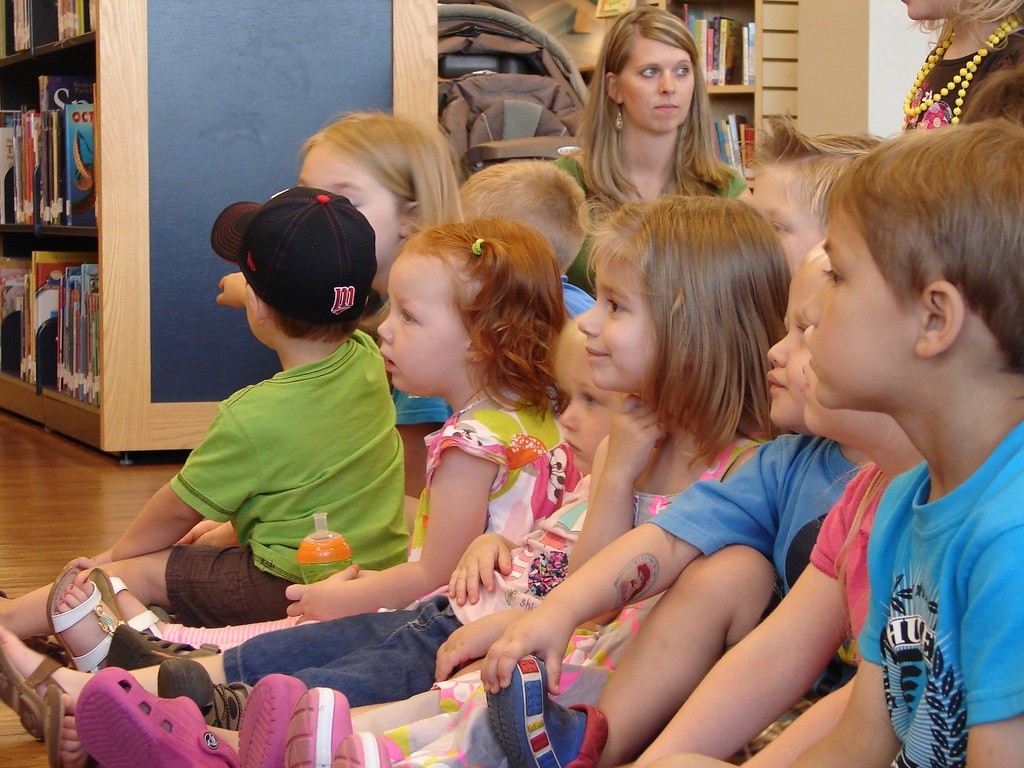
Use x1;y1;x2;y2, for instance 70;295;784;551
437;0;590;181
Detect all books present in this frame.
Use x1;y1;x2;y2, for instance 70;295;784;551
0;0;101;407
712;113;756;181
682;4;756;87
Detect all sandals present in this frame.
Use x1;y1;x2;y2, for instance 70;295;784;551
106;624;221;671
0;625;63;741
157;656;249;731
238;674;306;768
76;667;238;768
89;567;163;640
46;565;113;674
42;684;98;768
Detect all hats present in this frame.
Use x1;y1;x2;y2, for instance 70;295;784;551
210;187;378;325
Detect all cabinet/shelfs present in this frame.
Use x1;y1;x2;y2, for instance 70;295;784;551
0;0;871;460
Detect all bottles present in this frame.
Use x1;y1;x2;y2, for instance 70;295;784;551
297;510;352;587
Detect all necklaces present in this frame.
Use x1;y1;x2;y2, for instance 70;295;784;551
903;13;1022;131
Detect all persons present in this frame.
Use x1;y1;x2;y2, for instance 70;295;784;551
551;10;755;303
897;0;1024;133
1;112;1024;767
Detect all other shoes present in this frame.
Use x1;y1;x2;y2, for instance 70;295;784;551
332;729;391;768
485;655;607;768
285;688;352;768
